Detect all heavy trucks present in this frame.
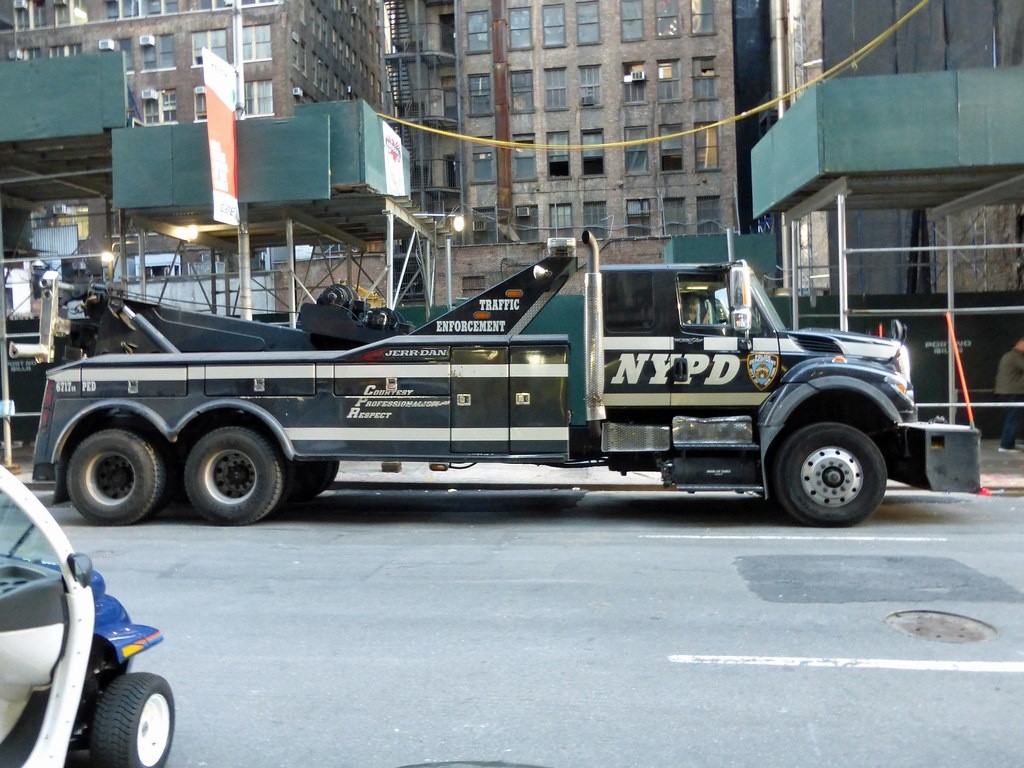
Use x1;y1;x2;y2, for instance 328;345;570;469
20;228;985;529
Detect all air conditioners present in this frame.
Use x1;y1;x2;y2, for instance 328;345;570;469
99;40;114;50
140;36;154;45
8;49;22;59
14;0;27;8
348;86;352;93
517;207;530;216
582;96;593;106
376;1;381;8
292;87;303;96
630;71;645;80
142;89;157;99
376;20;381;26
52;203;67;215
195;87;204;93
351;6;357;15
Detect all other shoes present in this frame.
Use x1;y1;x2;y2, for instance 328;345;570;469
998;447;1022;454
1015;438;1024;444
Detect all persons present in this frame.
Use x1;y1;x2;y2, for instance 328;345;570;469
682;293;710;326
994;339;1024;454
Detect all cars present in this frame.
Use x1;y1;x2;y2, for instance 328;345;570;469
0;462;180;768
